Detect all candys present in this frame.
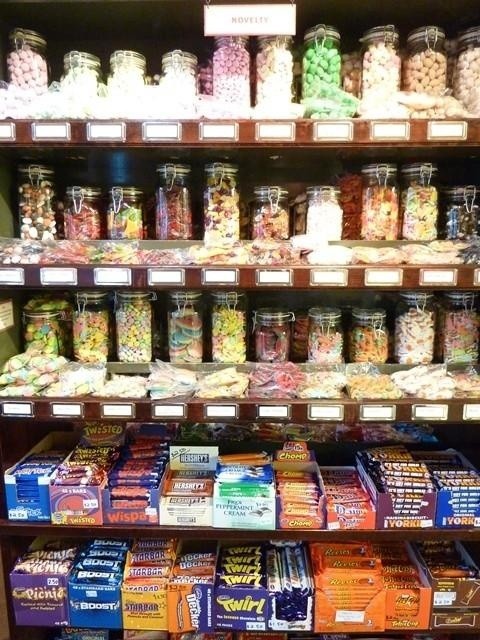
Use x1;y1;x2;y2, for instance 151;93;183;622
0;295;480;400
0;171;480;263
1;38;479;120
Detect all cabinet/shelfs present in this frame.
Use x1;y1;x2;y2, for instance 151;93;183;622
0;118;480;632
